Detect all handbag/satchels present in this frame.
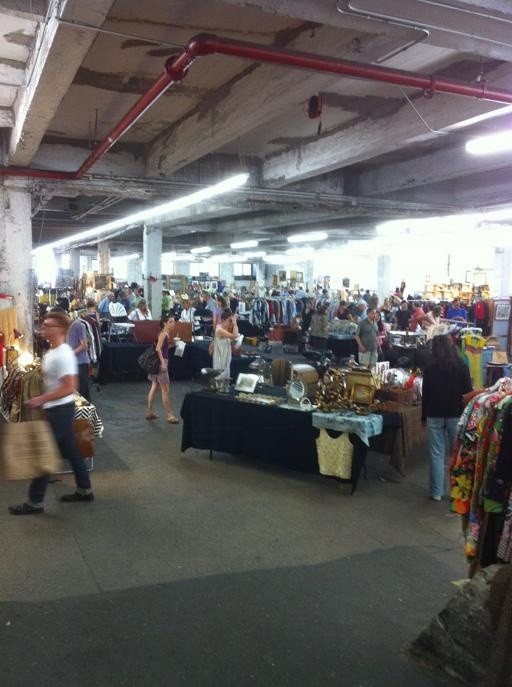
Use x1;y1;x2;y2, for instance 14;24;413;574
1;420;63;480
71;419;95;458
137;345;160;375
208;339;237;355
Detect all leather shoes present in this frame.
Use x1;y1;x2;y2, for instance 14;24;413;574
61;492;94;502
8;502;44;513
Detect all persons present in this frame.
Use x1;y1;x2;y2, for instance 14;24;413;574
8;312;94;515
53;281;243;423
299;285;490;364
423;333;470;501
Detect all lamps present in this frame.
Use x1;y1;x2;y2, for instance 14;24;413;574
7;327;26;349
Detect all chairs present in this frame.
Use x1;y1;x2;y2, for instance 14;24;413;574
106;298;140;345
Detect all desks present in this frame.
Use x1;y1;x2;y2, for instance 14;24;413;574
57;388;106;475
95;321;432;497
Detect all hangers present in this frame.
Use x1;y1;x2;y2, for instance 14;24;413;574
460;375;512;411
